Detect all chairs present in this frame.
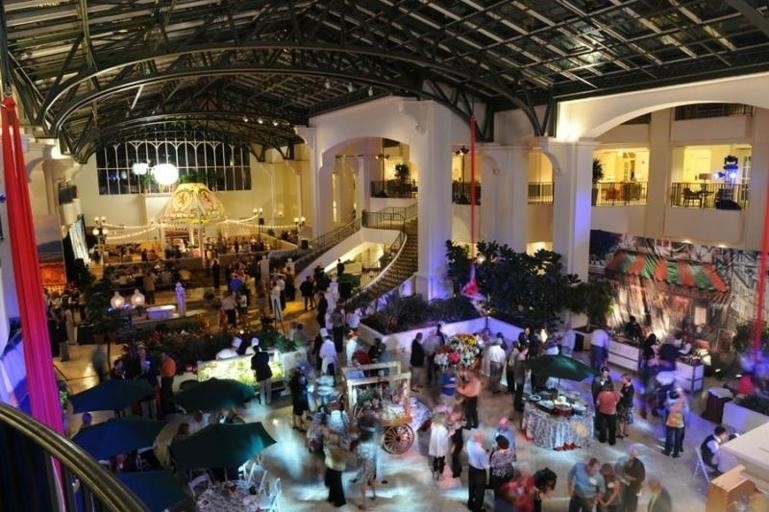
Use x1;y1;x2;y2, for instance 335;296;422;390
682;186;734;209
693;444;715;484
187;457;286;512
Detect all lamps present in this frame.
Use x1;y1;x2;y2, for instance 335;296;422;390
109;288;147;318
93;215;106;225
373;153;392;161
92;226;109;239
241;113;280;127
294;216;306;227
322;76;375;98
253;207;263;216
454;147;469;157
698;173;712;192
714;171;725;180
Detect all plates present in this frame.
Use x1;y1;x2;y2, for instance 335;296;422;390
528;392;586;415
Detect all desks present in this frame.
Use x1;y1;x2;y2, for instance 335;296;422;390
523;390;595;451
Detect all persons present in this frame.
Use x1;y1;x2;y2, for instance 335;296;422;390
43;233;768;511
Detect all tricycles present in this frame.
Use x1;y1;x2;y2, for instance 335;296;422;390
305;360;415;455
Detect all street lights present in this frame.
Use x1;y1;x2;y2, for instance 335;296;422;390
293;216;307;242
92;228;109;279
94;216;106;244
253;208;263;241
107;288;145;358
